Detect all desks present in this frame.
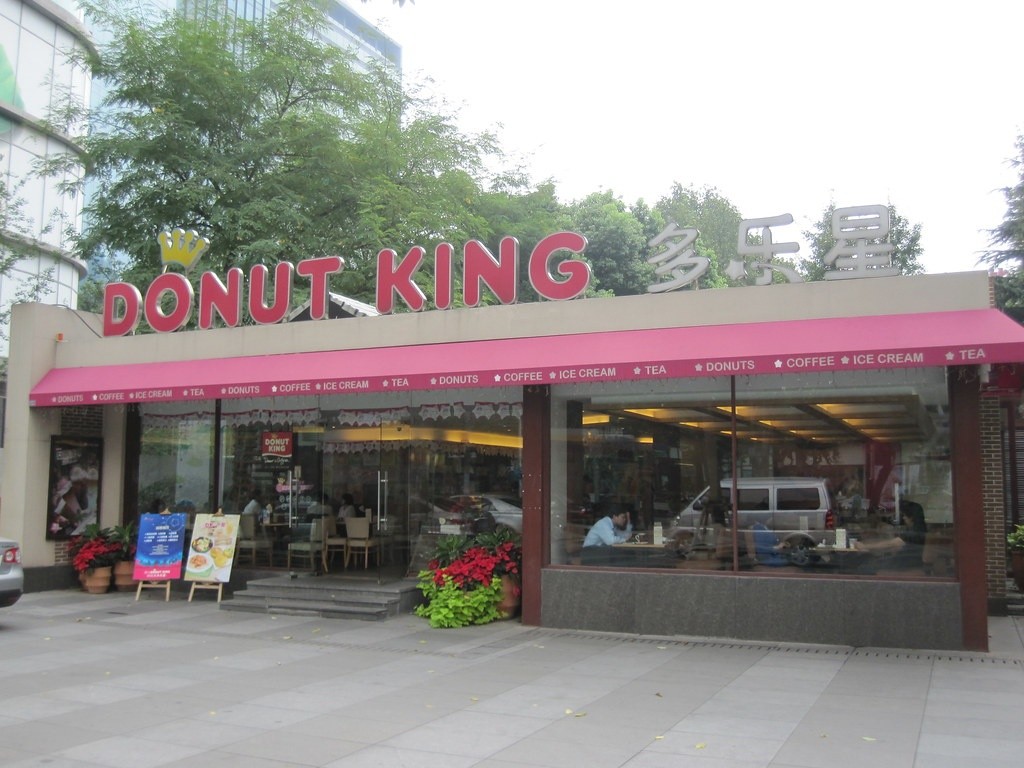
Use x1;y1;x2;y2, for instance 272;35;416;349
259;521;316;567
611;542;671;569
809;545;870;574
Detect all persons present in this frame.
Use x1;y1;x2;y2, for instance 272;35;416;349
239;486;278;552
306;491;336;569
335;490;357;538
582;506;633;566
750;505;807;573
850;499;927;578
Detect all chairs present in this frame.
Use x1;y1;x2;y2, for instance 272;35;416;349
233;509;396;574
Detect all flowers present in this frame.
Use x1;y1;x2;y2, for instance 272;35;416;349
66;519;138;576
413;525;522;629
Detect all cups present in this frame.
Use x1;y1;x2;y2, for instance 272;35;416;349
849;538;858;549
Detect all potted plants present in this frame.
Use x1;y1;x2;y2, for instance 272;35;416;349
1006;523;1024;587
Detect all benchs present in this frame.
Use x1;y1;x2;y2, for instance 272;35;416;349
678;524;727;571
561;522;608;566
743;529;805;574
874;531;951;577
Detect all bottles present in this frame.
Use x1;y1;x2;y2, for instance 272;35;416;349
835;526;846;549
654;520;663;546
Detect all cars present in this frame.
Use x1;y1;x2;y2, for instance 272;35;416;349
350;491;522;542
0;536;25;607
265;500;320;531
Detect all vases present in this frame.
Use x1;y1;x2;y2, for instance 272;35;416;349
78;571;89;591
83;566;112;594
493;575;522;620
113;561;139;592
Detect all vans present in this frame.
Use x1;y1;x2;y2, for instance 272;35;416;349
668;476;840;568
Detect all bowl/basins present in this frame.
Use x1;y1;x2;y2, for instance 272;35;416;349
186;555;213;576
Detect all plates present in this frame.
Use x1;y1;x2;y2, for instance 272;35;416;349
192;537;214;553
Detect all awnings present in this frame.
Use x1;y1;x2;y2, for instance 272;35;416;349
28;309;1024;399
